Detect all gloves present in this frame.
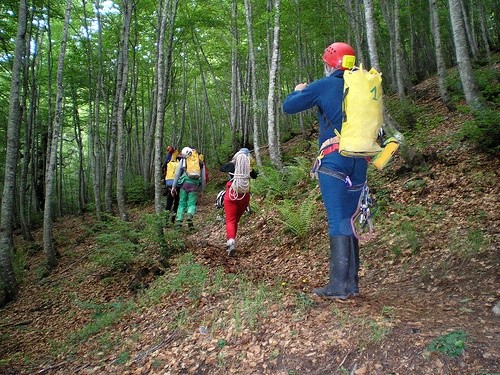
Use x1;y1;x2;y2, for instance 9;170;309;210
171;188;177;197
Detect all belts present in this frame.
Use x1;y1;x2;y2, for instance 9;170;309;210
322;143;371;161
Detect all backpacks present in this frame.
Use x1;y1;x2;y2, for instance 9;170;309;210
338;64;400;172
166;161;180;187
186;149;201;179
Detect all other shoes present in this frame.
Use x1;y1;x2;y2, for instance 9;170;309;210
187;218;194;228
226;239;236;256
179;225;182;228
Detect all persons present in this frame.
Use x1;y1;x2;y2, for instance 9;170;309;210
282;42;370;300
162;146;180;225
171;146;205;230
218;148;257;257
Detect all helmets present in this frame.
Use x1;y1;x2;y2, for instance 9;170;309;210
181;146;190;154
241;146;250;154
319;42;356;69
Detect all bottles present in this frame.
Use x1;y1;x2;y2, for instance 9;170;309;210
371;132;403;169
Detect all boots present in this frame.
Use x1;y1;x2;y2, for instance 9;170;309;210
312;233;361;296
